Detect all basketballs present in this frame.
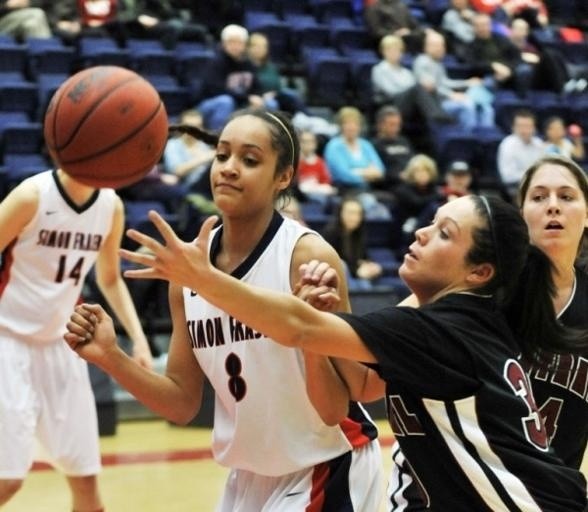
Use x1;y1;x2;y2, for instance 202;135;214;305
44;66;168;187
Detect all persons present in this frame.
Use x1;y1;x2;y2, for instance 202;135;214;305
355;1;588;260
396;153;587;472
164;107;391;280
0;0;215;51
62;106;387;512
117;194;587;511
198;25;281;127
1;170;156;510
